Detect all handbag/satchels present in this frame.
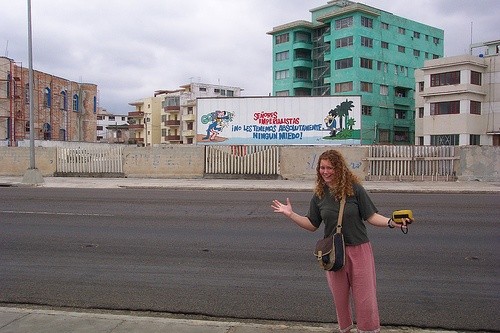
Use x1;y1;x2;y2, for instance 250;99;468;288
313;233;345;272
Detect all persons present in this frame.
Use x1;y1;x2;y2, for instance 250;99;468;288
271;149;411;333
